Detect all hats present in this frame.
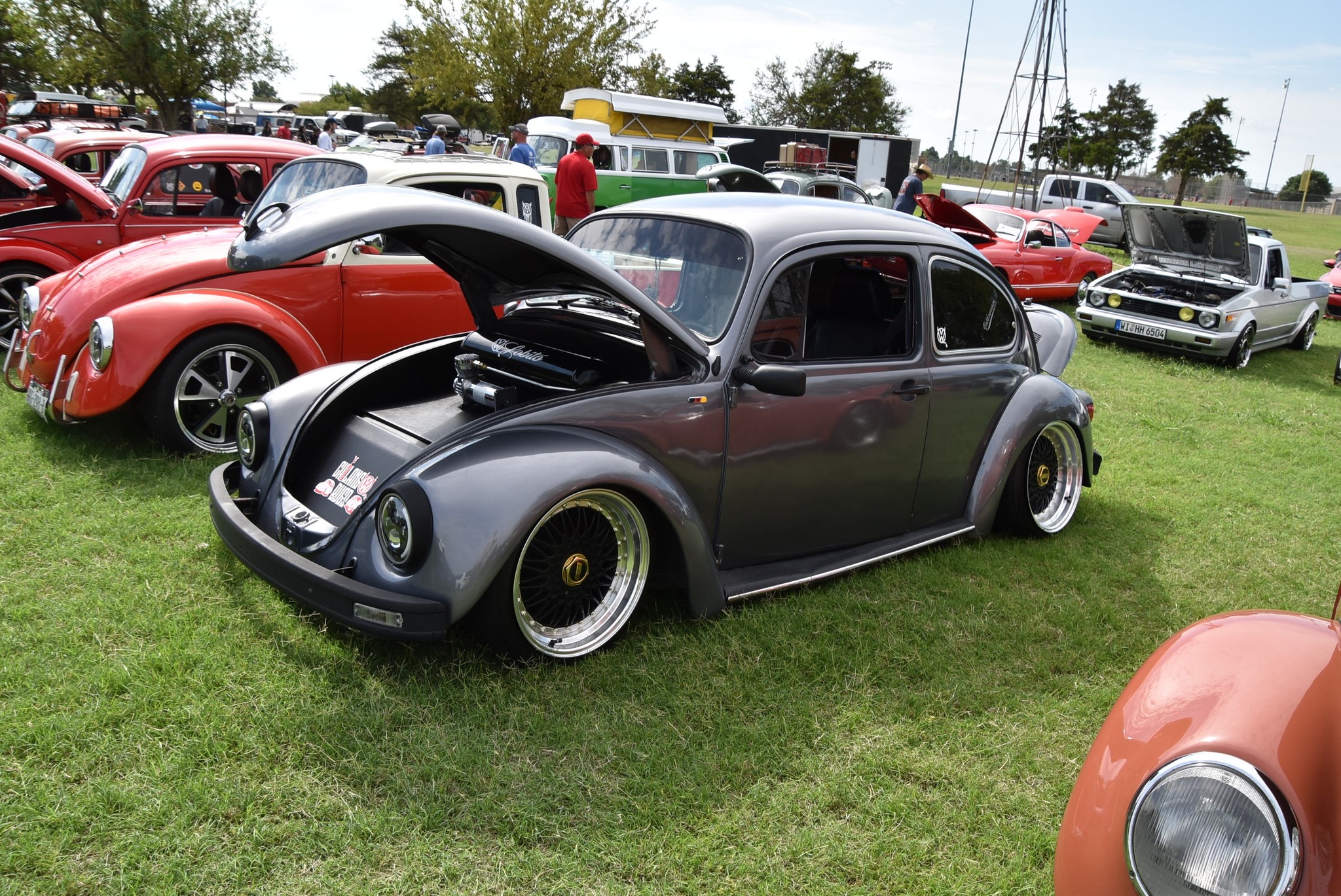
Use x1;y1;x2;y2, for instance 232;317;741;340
326;117;341;126
913;164;934;179
437;125;448;134
285;120;292;124
575;133;599;146
508;123;529;135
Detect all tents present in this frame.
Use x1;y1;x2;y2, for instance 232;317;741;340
161;97;226;122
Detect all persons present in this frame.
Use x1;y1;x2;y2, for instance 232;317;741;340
892;163;934;215
309;126;321;146
1186;193;1203;203
196;114;210;134
1244;198;1249;207
1228;198;1233;206
552;134;599;241
846;149;857;182
262;120;273;137
179;109;194;132
425;125;448;157
297;125;309;145
143;104;156;116
1128;187;1178;200
317;117;342;152
509;123;536;170
277;120;292;140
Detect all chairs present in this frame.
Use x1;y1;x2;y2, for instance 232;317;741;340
805;264;906;360
199;167;263;218
74;154;92;173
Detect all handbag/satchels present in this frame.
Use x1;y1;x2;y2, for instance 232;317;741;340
292;136;301;142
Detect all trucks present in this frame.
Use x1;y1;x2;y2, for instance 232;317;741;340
939;175;1138;254
242;116;361;147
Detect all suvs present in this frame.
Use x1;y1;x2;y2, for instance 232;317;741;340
336;113;487;158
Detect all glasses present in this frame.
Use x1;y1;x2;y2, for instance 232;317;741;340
510;131;513;133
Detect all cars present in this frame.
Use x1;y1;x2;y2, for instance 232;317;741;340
1319;260;1341;320
1053;581;1341;896
0;115;346;359
1246;225;1278;287
209;183;1102;665
695;162;892;210
861;194;1113;306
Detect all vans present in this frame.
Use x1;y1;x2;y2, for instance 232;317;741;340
491;87;732;249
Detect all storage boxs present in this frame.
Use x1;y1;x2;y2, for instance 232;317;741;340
780;142;827;169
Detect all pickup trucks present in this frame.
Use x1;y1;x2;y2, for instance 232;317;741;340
1075;203;1329;371
5;153;685;460
